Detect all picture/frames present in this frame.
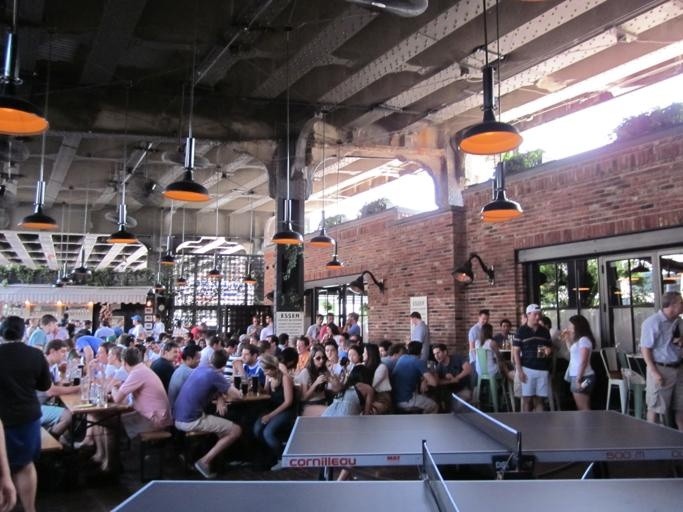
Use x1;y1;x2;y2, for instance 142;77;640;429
143;306;153;329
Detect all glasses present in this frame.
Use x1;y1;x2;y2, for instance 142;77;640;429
312;356;326;361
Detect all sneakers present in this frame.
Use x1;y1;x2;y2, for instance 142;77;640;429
271;460;283;473
195;460;216;479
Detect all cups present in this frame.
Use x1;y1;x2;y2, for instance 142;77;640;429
233;373;259;397
502;334;515;350
77;375;107;407
73;377;79;385
558;329;568;342
537;345;545;357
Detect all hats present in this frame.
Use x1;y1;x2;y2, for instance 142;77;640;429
526;304;541;313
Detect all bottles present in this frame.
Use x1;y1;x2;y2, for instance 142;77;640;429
580;378;592;389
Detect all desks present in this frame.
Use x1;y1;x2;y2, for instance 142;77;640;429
96;434;681;512
211;392;274;467
281;392;682;478
55;391;137;486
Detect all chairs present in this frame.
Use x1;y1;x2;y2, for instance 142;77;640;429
472;345;663;424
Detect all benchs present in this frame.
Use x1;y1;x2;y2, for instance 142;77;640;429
38;422;65;453
139;431;171;482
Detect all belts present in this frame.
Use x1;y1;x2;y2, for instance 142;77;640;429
654;361;681;368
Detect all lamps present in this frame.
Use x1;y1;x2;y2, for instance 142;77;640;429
1;1;345;285
567;258;677;295
478;3;523;224
344;269;386;296
456;1;523;159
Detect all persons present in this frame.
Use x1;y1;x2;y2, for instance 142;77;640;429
508;304;570;413
468;310;490;388
563;314;596;411
639;292;683;432
0;309;472;512
494;318;513;345
474;324;502;378
